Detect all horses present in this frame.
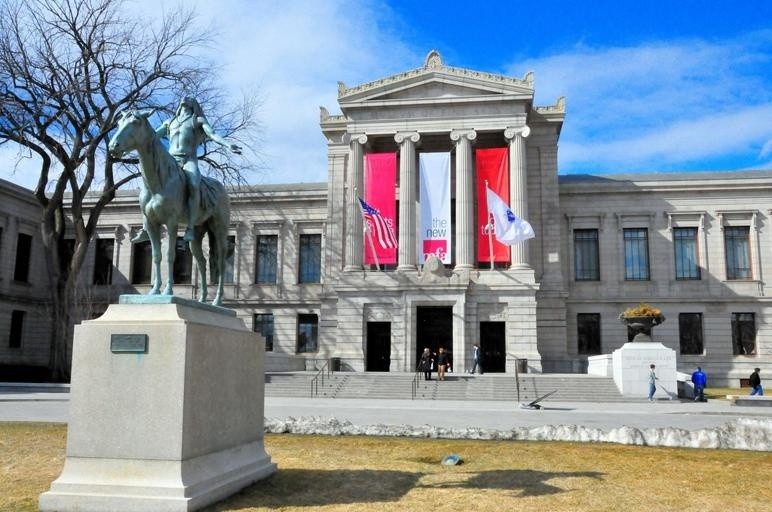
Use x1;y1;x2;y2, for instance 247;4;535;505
106;104;231;305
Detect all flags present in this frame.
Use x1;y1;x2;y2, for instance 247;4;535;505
353;186;398;250
484;177;536;249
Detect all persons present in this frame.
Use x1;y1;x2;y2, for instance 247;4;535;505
690;365;707;402
746;367;762;396
645;362;660;403
432;346;450;381
128;93;245;243
468;344;483;374
421;346;435;381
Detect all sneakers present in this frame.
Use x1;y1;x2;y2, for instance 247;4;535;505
425;371;484;381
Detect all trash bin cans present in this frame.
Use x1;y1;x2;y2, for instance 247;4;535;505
330;357;341;371
516;358;527;373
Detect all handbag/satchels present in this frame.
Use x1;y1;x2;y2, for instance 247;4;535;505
430;362;433;370
